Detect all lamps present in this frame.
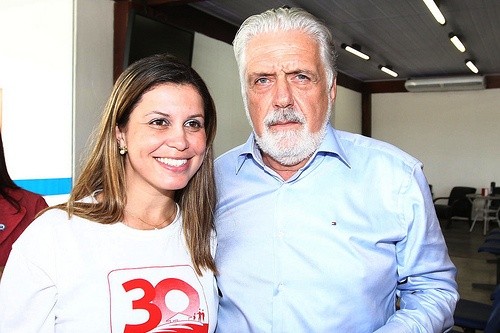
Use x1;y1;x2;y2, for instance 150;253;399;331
341;43;371;62
378;64;400;78
448;31;468;54
422;0;448;27
464;58;480;74
405;74;487;92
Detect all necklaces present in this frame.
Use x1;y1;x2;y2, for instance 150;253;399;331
117;200;178;230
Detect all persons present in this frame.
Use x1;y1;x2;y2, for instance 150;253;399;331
0;52;221;333
0;129;49;280
211;6;462;332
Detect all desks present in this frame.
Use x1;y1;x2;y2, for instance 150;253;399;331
465;193;500;236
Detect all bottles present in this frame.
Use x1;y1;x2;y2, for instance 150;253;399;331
485;188;489;196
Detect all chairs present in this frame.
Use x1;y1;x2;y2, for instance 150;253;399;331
446;238;500;333
433;186;477;229
